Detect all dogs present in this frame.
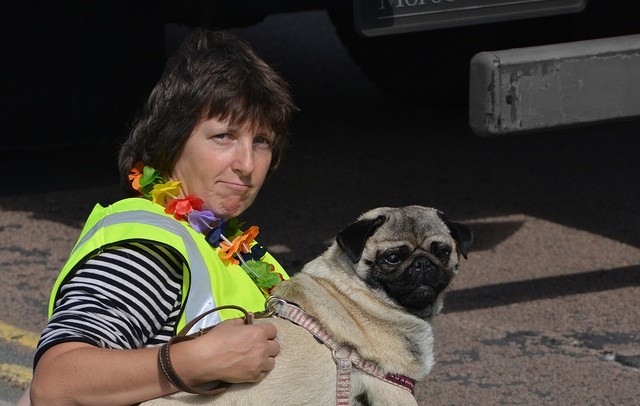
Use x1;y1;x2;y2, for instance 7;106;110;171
139;205;474;406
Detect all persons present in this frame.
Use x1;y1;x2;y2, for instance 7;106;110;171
17;32;300;406
